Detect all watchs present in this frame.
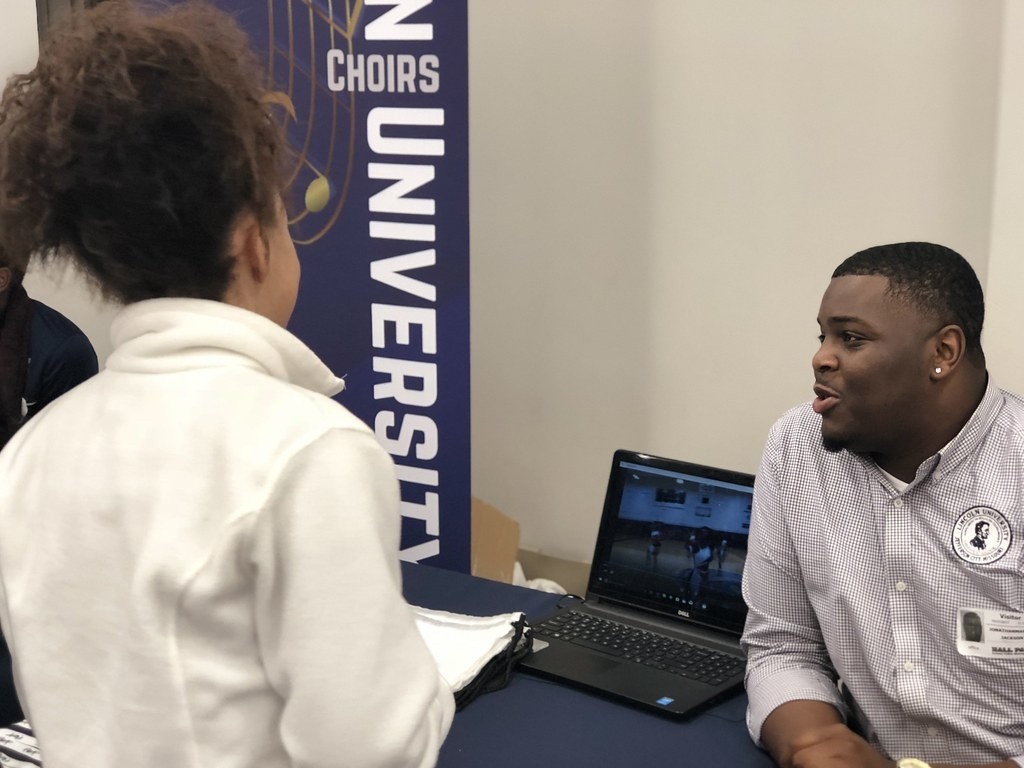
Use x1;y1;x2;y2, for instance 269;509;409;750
896;757;932;768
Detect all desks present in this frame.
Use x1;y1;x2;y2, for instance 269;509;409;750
396;555;857;768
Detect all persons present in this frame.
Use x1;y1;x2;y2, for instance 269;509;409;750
964;613;982;641
739;240;1024;768
644;518;729;601
0;1;457;768
1;231;100;729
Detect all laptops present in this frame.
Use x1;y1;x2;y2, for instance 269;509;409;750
516;449;756;722
